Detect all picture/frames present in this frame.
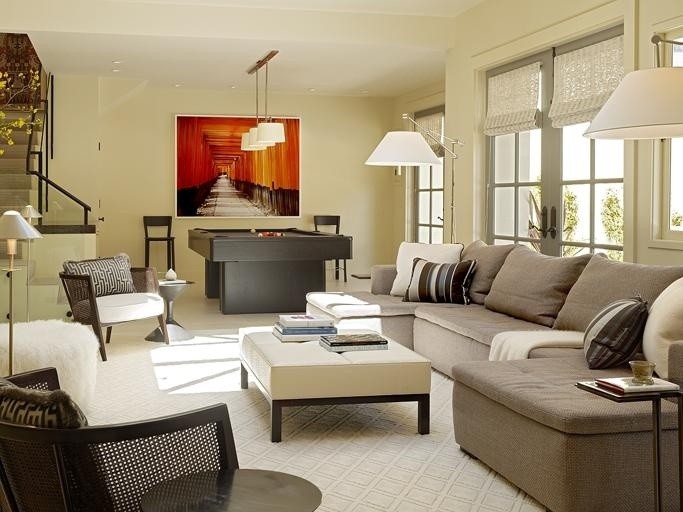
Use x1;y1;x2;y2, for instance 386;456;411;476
173;115;301;218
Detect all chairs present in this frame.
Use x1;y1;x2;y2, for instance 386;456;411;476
141;211;176;273
314;216;348;278
0;367;237;511
59;258;171;359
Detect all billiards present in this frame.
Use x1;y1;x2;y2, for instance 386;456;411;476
250;228;287;238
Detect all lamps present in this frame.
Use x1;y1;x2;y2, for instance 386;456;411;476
241;51;286;152
19;204;43;323
583;34;682;139
358;112;460;177
0;210;45;375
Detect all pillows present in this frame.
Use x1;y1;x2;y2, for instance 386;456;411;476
0;383;118;510
482;244;594;327
389;241;463;297
454;240;517;305
551;253;682;332
582;292;646;368
63;253;136;305
401;256;477;307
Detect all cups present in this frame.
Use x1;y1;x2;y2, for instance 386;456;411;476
629;361;656;385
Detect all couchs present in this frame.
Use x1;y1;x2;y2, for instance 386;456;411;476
304;236;682;512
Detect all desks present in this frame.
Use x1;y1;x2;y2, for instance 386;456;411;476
578;377;681;511
188;225;351;316
143;468;322;512
149;280;197;345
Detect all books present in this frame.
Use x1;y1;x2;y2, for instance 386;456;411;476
271;313;388;353
594;377;680;395
